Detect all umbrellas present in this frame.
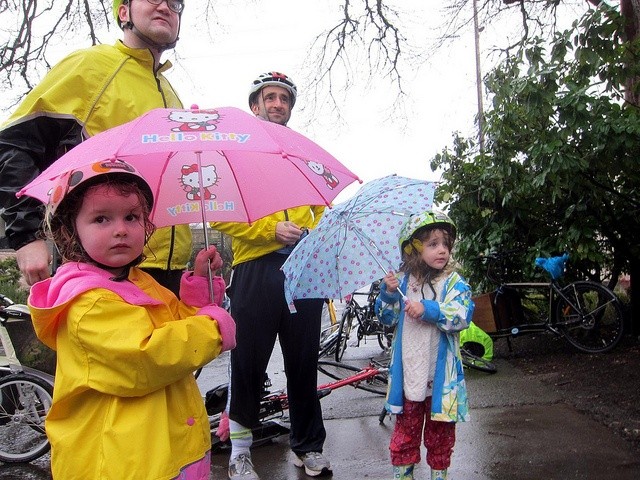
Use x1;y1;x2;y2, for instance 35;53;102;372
281;174;446;322
16;105;363;304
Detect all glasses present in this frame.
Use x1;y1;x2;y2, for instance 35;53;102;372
148;0;185;14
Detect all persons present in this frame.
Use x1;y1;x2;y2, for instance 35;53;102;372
1;1;193;297
210;72;333;479
374;211;475;479
27;159;236;480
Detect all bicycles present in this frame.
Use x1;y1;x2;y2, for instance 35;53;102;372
195;358;391;448
335;280;396;362
318;322;359;359
471;250;625;354
460;345;497;374
0;293;55;465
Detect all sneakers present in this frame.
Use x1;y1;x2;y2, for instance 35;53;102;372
293;451;333;476
227;450;260;480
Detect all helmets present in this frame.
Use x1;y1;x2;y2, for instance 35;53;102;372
248;71;297;109
44;158;154;232
111;1;129;29
396;209;457;260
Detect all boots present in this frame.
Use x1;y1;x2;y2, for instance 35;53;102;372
392;464;414;480
431;467;447;480
